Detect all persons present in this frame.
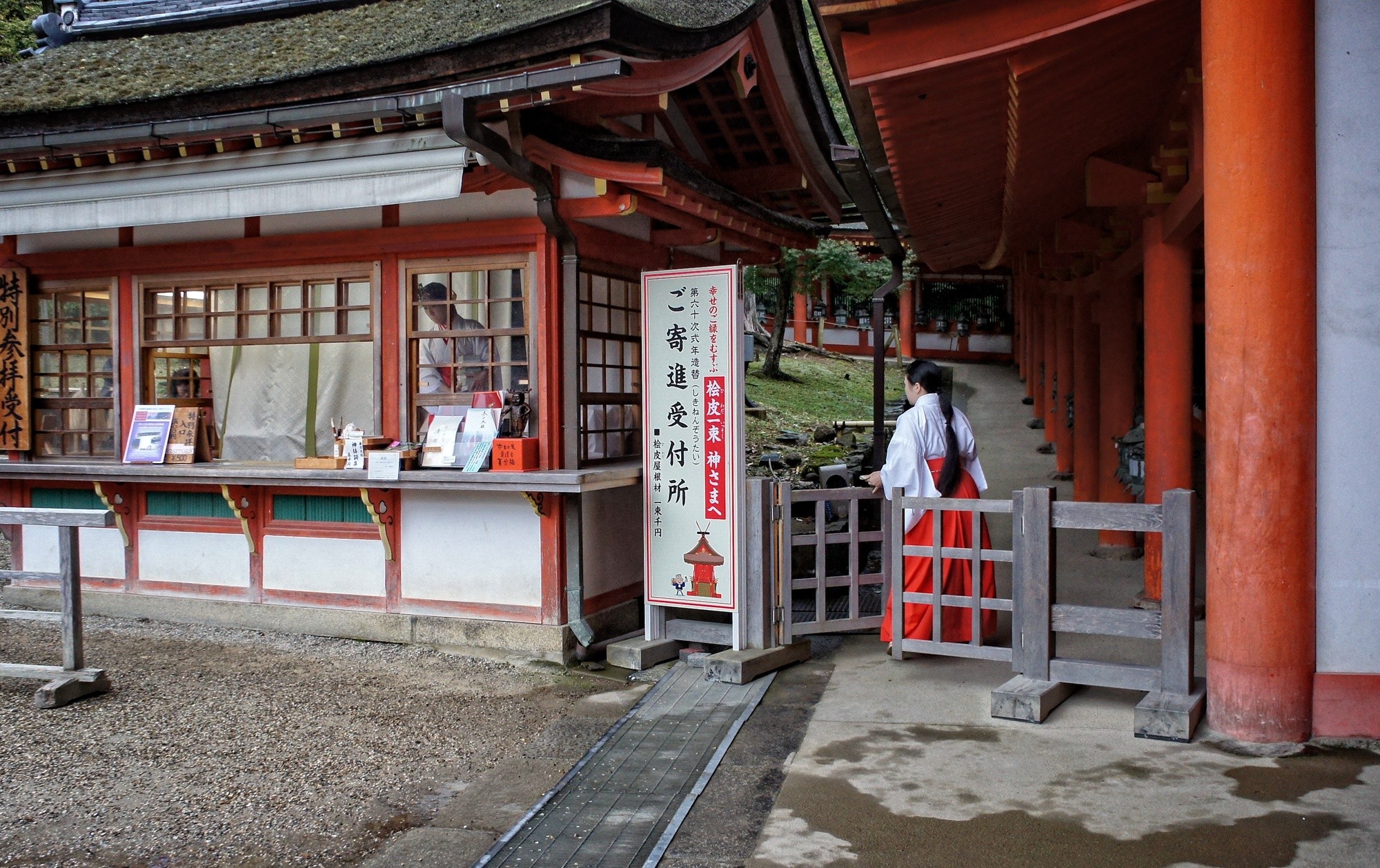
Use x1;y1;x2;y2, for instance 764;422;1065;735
169;369;199;400
416;282;498;415
97;359;114;426
865;359;998;655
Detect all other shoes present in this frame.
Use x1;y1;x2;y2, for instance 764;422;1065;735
887;641;893;655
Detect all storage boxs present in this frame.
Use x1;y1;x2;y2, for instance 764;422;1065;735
293;438;418;469
